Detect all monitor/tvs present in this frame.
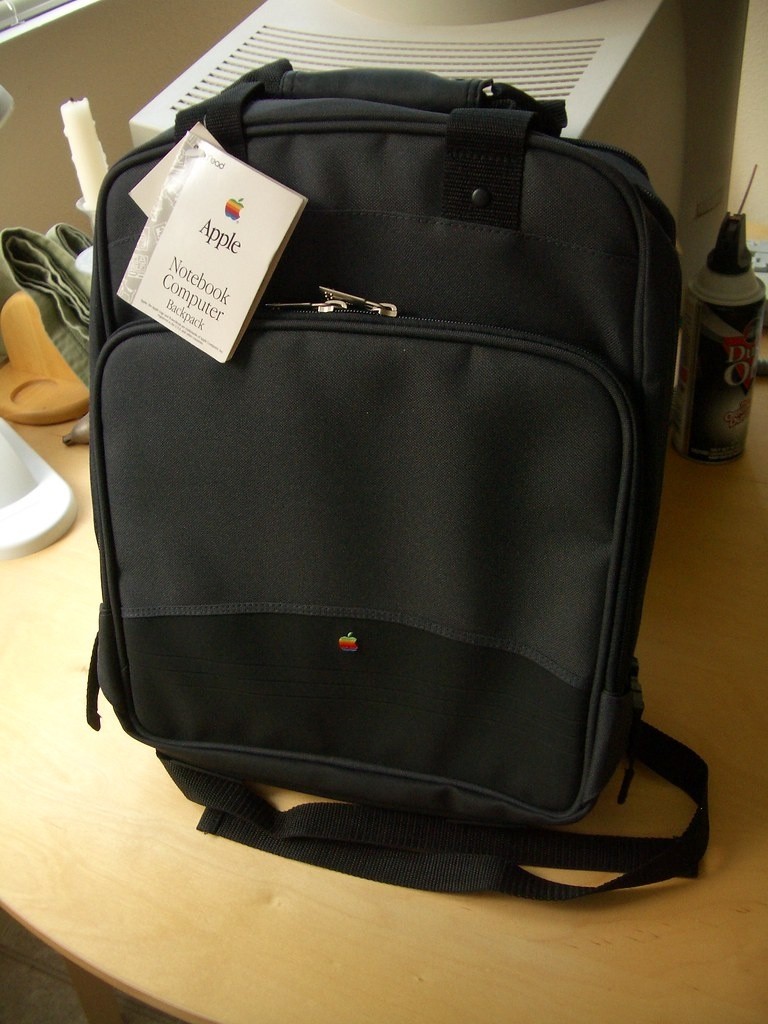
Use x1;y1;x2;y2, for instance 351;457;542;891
128;0;750;300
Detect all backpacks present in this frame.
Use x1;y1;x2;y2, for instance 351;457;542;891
83;59;714;898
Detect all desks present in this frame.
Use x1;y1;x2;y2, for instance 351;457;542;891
0;337;767;1023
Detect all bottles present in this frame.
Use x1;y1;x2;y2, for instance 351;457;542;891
671;211;766;465
